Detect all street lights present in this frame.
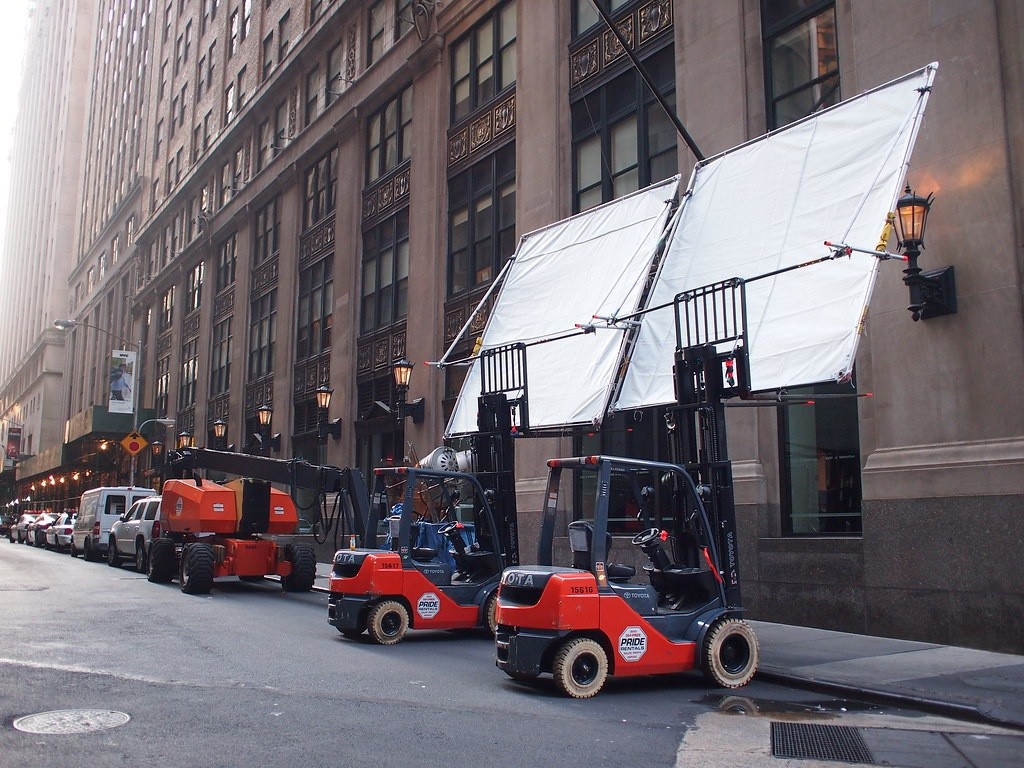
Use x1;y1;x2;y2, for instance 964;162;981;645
53;320;176;487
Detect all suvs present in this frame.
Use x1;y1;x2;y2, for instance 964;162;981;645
107;494;163;574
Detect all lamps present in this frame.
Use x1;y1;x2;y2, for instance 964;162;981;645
258;404;280;453
314;383;342;441
214;417;234;454
151;440;167;457
179;431;195;448
891;180;957;322
391;359;424;426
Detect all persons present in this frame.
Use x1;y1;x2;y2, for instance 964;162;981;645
110;363;131;401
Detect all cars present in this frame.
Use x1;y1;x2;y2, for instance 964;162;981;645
9;513;41;544
43;512;78;553
0;516;12;537
25;511;60;548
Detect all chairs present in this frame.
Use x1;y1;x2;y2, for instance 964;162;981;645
568;521;636;583
387;515;437;561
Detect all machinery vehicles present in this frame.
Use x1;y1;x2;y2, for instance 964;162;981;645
492;455;760;698
326;466;510;646
145;446;370;596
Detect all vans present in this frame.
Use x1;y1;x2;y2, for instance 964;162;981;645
69;486;157;561
